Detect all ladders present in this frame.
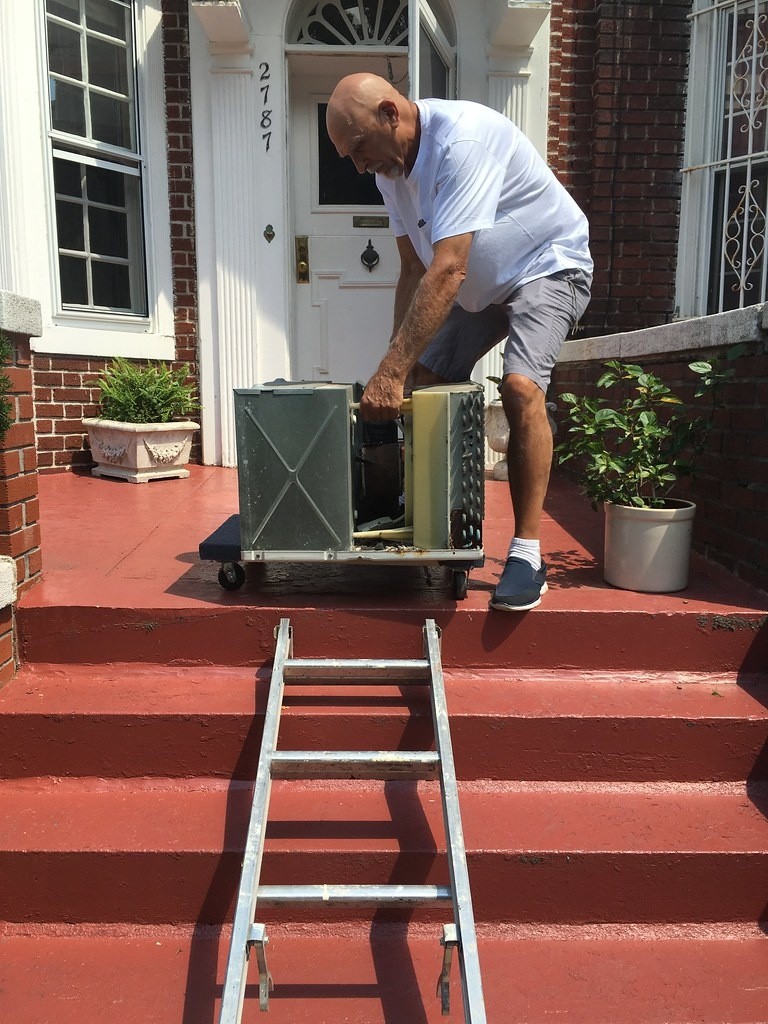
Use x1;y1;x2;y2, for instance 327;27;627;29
218;617;487;1024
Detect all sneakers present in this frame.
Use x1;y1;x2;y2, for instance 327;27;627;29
489;556;548;611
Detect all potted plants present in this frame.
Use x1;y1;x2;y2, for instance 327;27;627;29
553;346;744;594
82;355;204;485
485;353;559;482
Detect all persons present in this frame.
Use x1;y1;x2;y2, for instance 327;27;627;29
325;72;594;612
